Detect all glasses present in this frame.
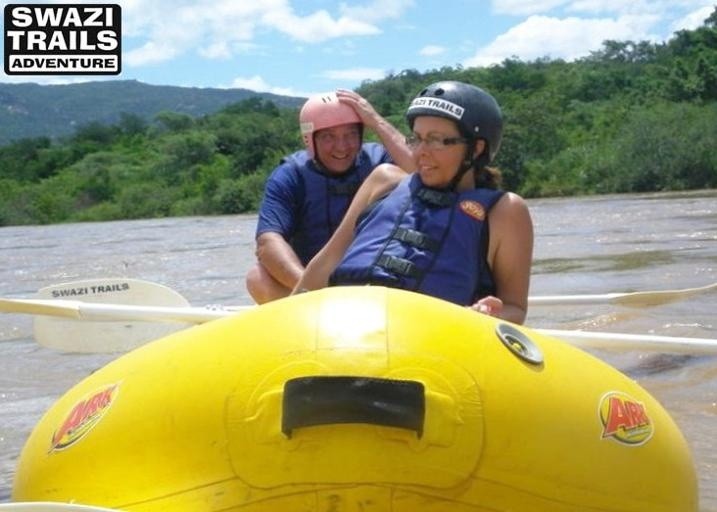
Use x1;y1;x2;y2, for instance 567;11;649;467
405;129;461;150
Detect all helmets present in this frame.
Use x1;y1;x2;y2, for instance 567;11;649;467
405;79;505;165
298;90;366;162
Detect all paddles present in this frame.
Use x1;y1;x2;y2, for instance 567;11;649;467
0;297;717;356
32;277;717;354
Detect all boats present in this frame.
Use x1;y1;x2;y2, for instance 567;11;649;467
9;284;700;512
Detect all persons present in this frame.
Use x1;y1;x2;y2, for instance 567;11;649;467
244;89;417;308
288;80;535;326
